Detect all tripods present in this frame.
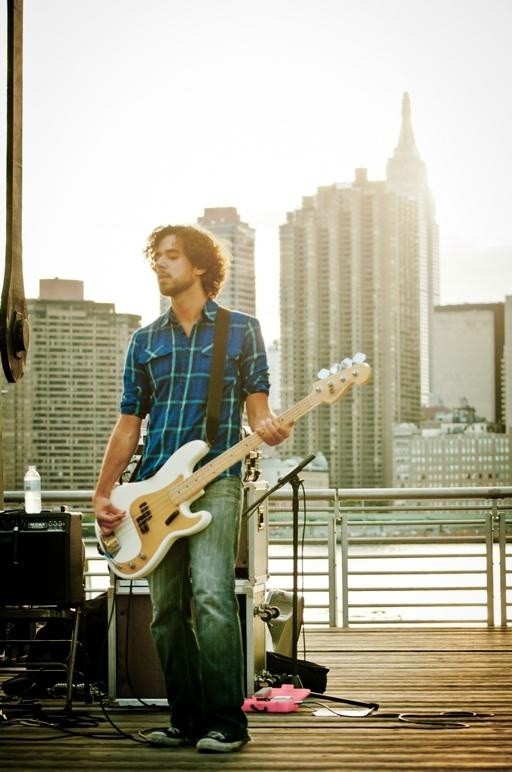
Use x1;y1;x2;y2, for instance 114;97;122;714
242;453;379;711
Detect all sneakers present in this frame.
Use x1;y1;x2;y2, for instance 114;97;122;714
196;729;250;752
147;727;196;746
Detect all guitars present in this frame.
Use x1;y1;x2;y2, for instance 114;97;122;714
94;353;371;579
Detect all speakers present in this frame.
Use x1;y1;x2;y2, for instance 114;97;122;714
0;511;85;608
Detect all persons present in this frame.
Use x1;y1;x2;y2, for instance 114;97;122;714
92;224;296;755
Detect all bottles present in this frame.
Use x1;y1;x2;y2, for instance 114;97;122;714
24;466;41;514
